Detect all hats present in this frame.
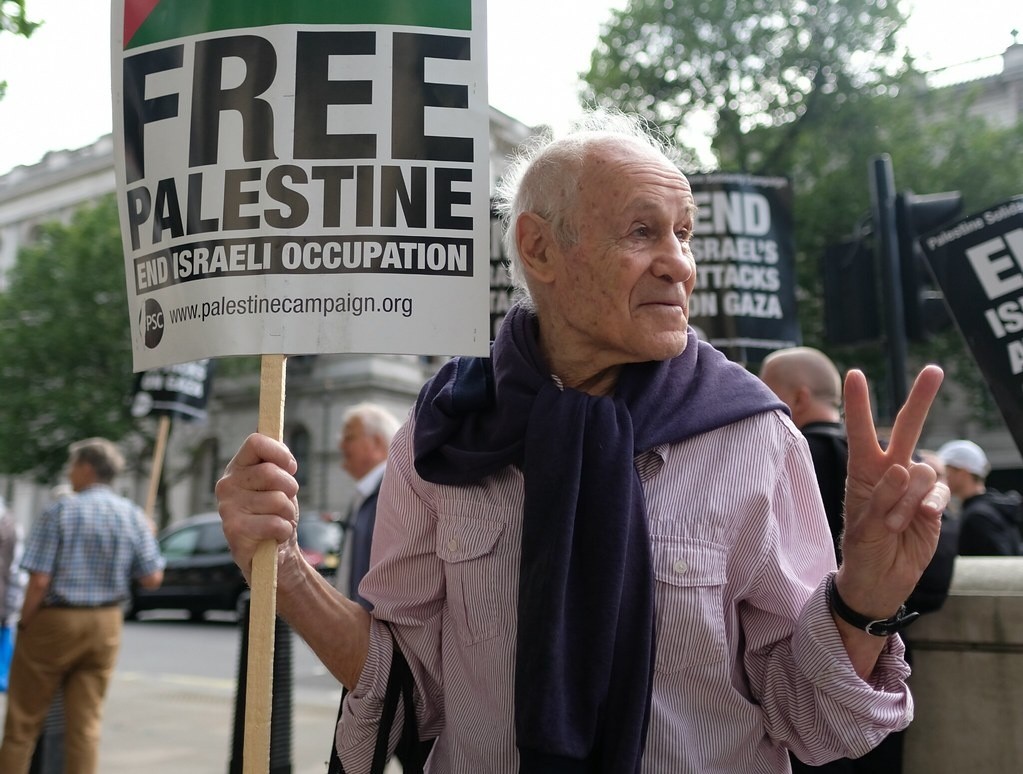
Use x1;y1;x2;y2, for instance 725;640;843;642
916;439;992;479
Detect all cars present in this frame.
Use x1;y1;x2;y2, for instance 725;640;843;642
120;511;342;624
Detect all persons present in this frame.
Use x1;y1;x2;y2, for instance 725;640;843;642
0;437;166;774
939;438;1023;556
760;347;953;774
335;402;401;612
214;129;953;774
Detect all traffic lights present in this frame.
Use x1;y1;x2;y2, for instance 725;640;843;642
897;184;970;343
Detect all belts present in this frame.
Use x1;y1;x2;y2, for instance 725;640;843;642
48;594;120;610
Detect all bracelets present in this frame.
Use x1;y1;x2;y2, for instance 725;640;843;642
831;574;920;637
